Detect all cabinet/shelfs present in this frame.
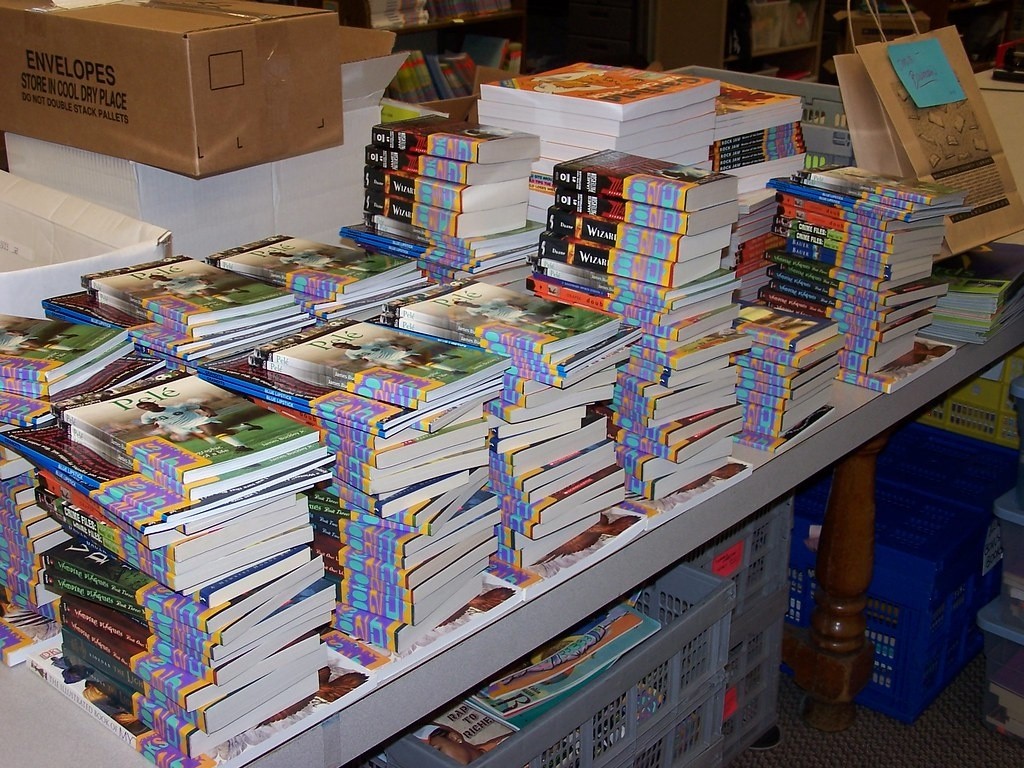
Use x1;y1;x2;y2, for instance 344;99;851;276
273;1;1024;117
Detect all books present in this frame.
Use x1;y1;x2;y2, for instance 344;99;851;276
339;114;546;294
766;163;1024;394
0;314;179;667
0;373;376;768
393;283;644;567
376;586;700;768
369;0;522;104
729;300;845;453
205;235;439;328
196;318;503;658
478;62;806;301
525;150;752;502
41;255;316;375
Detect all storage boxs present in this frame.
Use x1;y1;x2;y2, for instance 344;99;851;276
834;8;930;54
0;0;524;322
360;344;1024;768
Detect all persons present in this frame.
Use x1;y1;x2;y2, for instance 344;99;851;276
412;721;516;768
332;341;469;374
149;274;250;306
454;298;580;333
0;329;87;355
269;249;380;274
136;402;263;452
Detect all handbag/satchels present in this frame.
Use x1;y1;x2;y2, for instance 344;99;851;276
834;1;1023;260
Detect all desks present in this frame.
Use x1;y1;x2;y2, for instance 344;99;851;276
0;311;1024;768
